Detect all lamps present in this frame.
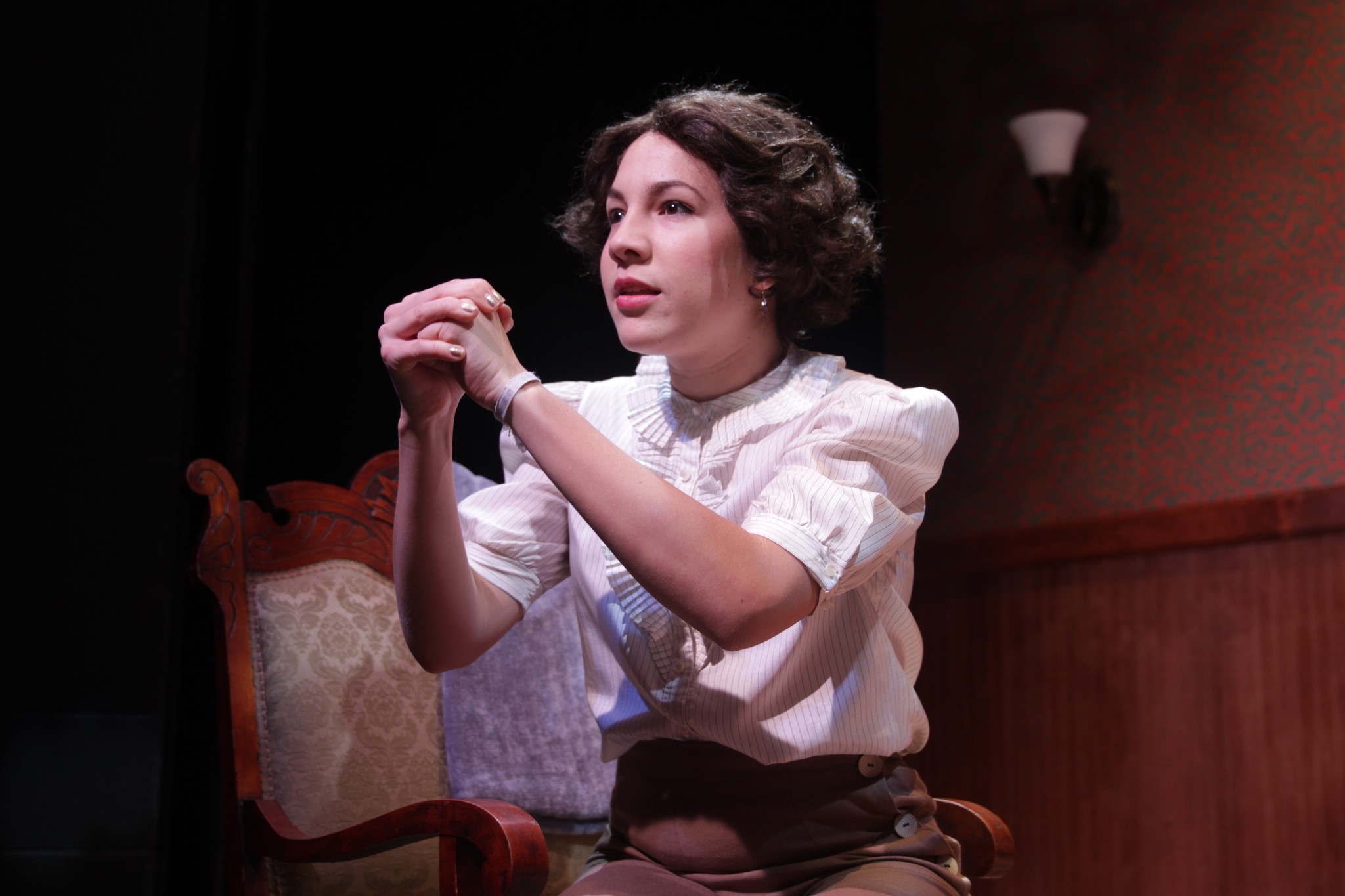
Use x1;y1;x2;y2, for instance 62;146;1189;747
1005;106;1130;269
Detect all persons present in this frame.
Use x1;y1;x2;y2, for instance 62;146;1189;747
378;86;972;896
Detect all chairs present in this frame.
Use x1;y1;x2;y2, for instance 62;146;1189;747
181;447;1021;896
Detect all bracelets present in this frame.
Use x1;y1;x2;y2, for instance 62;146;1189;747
494;371;541;423
